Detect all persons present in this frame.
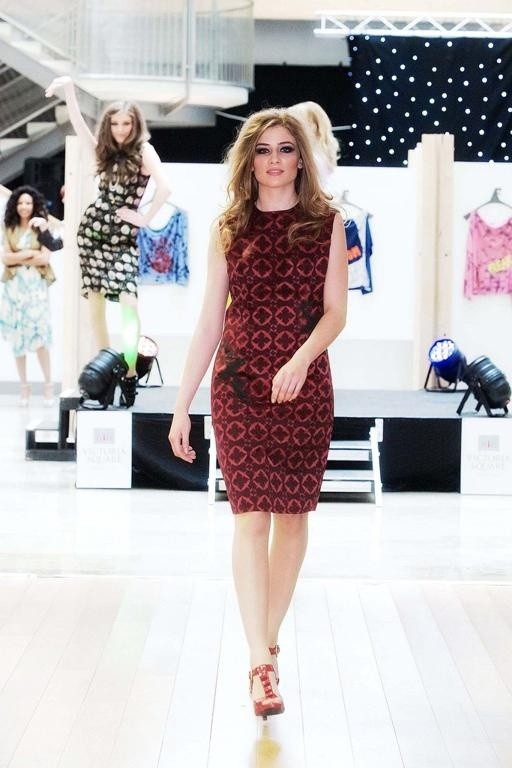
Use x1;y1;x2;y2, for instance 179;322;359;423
286;99;342;177
42;72;172;408
0;184;59;410
28;183;66;253
167;106;352;721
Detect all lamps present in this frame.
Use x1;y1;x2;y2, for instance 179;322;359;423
136;334;166;386
425;340;466;393
456;357;511;418
79;349;128;409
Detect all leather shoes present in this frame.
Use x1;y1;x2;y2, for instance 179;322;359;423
120;376;136;406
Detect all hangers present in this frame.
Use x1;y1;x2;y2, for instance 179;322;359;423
138;196;177;212
464;188;512;221
338;190;373;218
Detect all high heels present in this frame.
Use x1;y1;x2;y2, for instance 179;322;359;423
248;645;285;721
